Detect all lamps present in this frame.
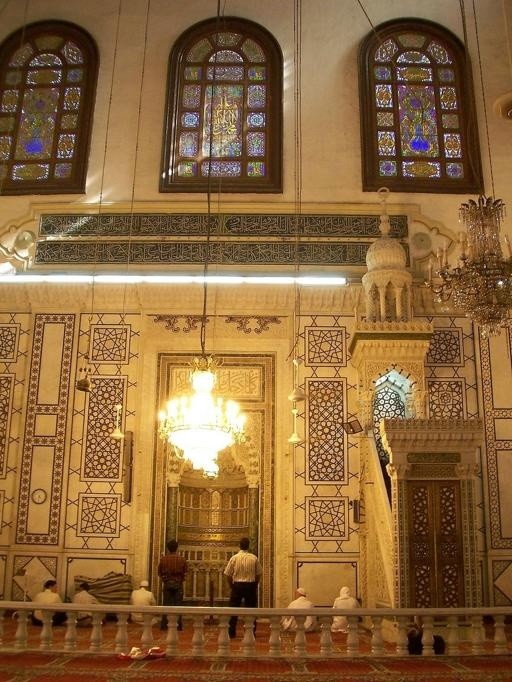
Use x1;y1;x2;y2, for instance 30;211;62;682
416;0;512;337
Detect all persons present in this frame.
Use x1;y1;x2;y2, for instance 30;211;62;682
29;580;65;626
72;582;108;627
281;586;316;631
127;579;156;623
157;543;188;630
223;538;264;639
330;586;363;633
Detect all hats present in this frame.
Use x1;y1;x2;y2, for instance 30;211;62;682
140;581;149;586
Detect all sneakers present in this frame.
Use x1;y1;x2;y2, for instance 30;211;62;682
229;630;235;635
177;624;182;629
160;625;167;629
